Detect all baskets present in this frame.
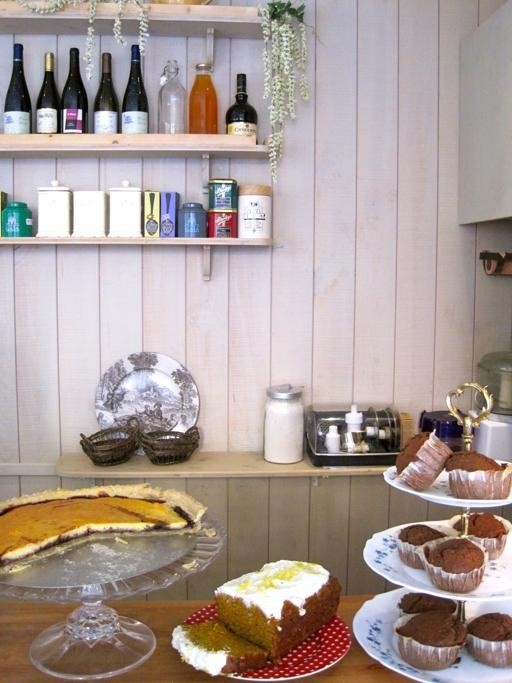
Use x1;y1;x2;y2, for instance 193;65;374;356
138;426;200;464
80;417;142;466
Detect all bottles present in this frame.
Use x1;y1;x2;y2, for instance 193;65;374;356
3;43;148;136
419;410;475;451
226;72;262;145
36;180;142;237
176;178;273;237
1;202;31;236
326;405;363;453
154;61;189;136
192;57;219;134
0;189;10;234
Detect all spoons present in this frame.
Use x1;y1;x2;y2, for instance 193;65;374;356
146;193;158;235
161;193;174;235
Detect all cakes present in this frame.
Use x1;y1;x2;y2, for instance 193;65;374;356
0;485;206;581
171;559;340;675
393;428;512;672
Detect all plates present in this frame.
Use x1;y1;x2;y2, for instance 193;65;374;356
383;459;512;507
94;351;200;455
352;586;510;682
362;519;512;599
180;601;352;679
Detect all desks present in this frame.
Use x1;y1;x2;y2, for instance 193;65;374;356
0;595;413;683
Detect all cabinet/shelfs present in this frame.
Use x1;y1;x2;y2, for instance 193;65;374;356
0;1;283;281
